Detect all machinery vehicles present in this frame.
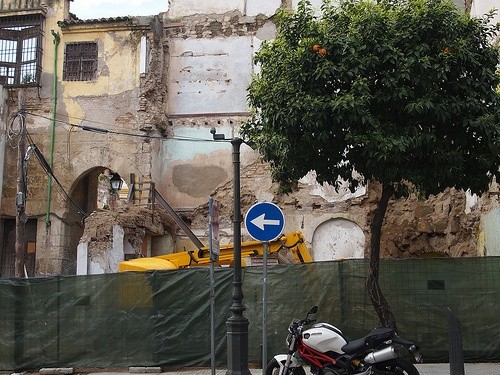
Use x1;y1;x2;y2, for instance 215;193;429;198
119;231;325;310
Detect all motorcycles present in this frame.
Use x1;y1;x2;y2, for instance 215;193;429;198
263;307;422;375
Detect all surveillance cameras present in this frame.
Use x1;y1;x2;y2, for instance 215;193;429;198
210;127;216;135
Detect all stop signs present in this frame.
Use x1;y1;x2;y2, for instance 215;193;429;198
212;201;219;240
